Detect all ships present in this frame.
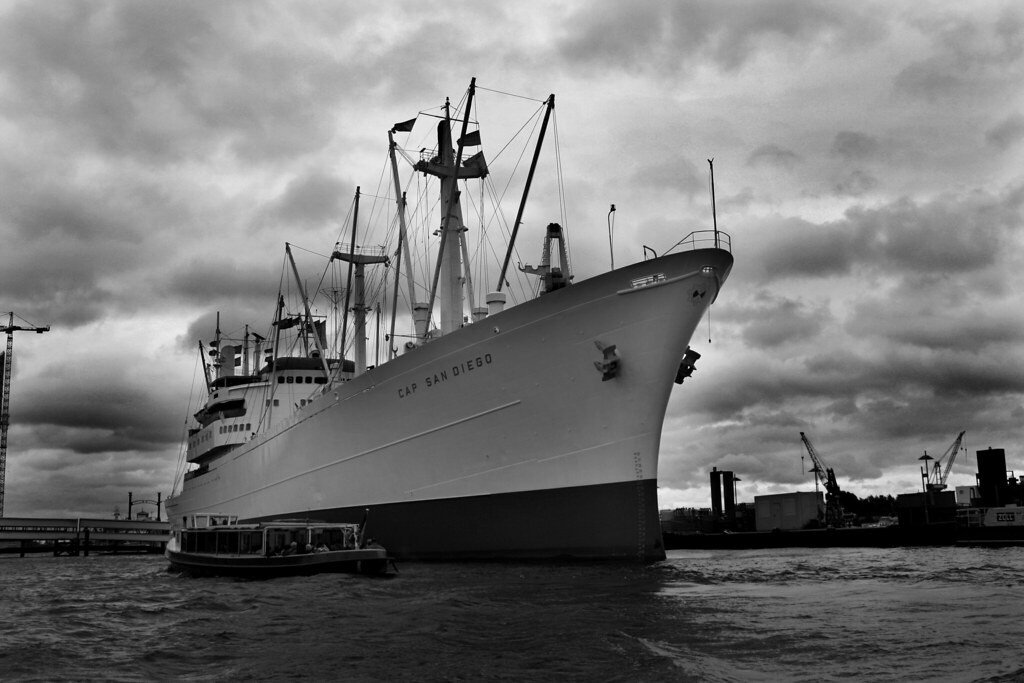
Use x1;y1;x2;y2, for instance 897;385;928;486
162;77;738;568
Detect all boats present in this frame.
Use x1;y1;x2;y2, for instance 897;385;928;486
167;506;398;579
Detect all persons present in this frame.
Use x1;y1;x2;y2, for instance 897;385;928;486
245;542;341;555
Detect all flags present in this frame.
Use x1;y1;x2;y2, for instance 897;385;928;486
457;129;481;147
395;118;416;132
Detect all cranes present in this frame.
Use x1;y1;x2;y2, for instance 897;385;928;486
937;427;967;487
798;431;835;491
0;309;51;520
